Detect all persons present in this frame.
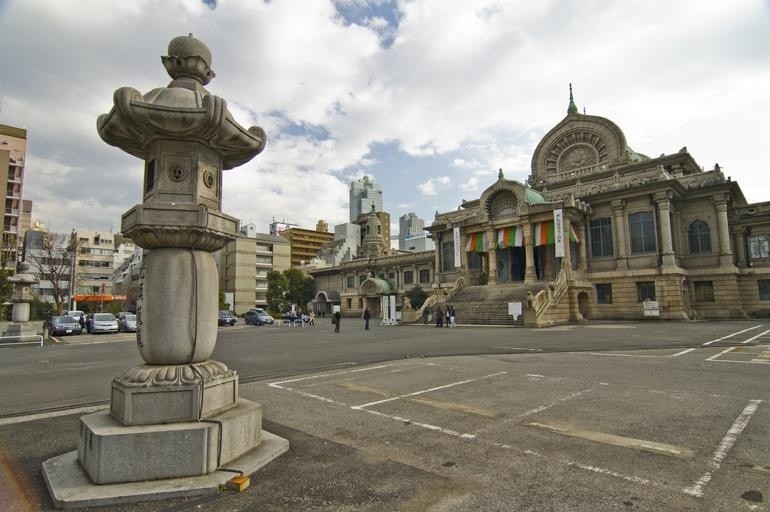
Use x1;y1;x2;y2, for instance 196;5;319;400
296;309;303;327
335;308;341;332
423;303;457;329
363;305;373;331
309;308;316;326
79;313;85;333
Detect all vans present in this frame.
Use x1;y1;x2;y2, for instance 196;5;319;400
245;308;274;326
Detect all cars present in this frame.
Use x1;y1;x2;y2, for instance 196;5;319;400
42;311;137;336
281;311;310;323
218;309;235;326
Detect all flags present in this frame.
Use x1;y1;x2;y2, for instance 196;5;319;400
568;222;583;244
463;220;556;254
